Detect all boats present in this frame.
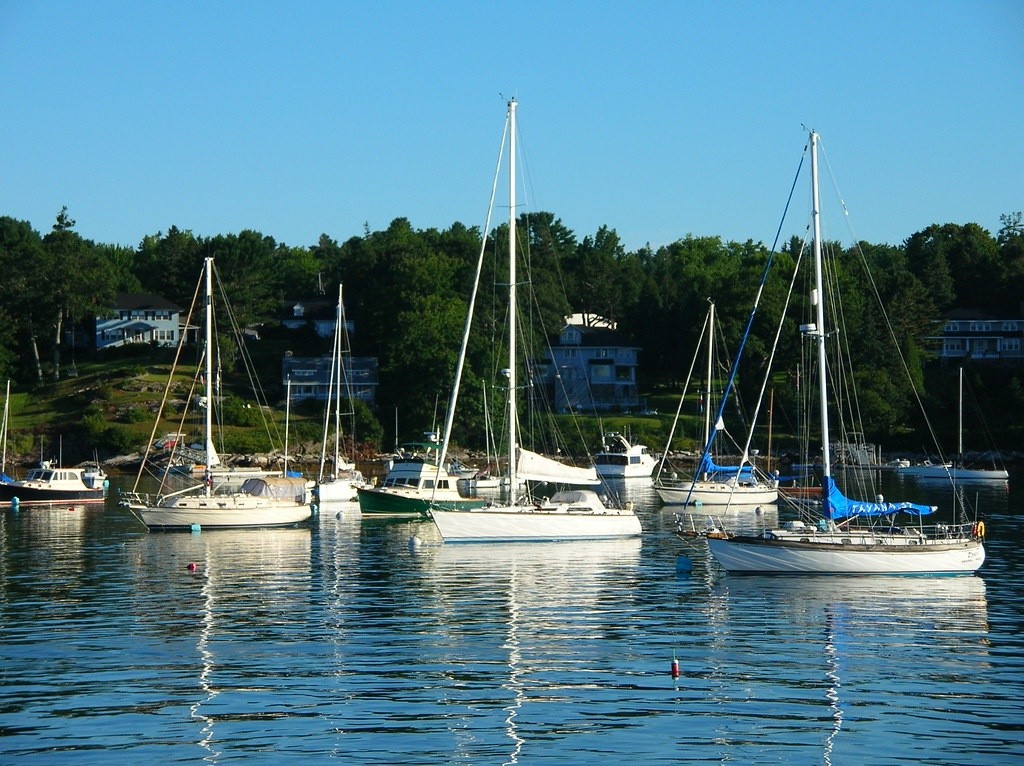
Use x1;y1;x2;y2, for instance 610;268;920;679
0;434;106;504
384;425;479;480
894;447;952;473
349;394;487;516
74;448;107;489
589;417;660;478
888;459;911;469
158;463;206;474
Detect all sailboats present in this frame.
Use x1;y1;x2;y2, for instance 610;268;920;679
305;284;377;504
117;257;313;531
651;297;779;505
465;379;526;488
0;380;15;483
920;367;1009;479
670;123;987;575
429;92;643;543
768;388;823;498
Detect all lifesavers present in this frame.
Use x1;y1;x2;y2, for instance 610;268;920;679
977;520;985;537
206;478;211;486
